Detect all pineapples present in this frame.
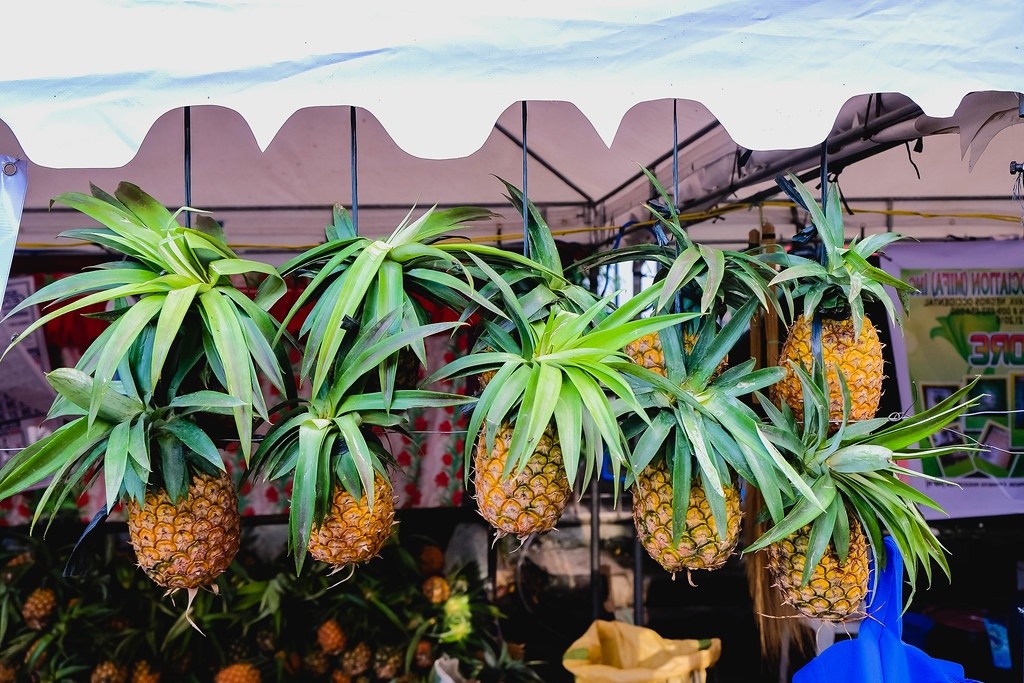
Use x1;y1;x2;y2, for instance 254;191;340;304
0;168;986;683
